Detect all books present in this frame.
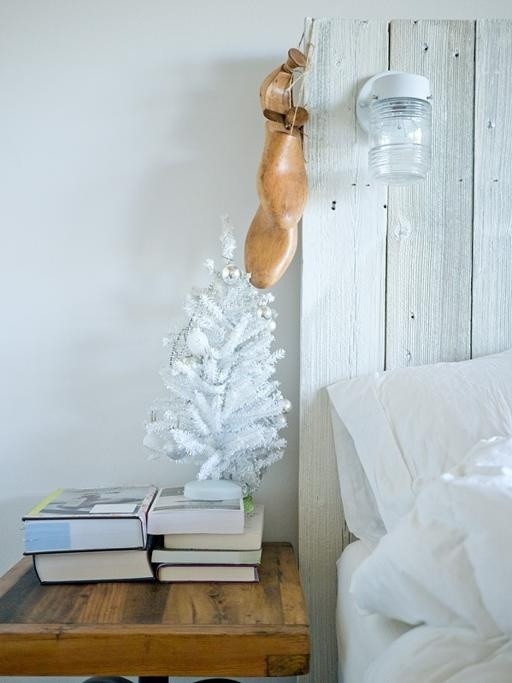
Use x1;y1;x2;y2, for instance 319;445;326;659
147;484;246;536
149;535;263;565
158;502;268;550
155;560;261;584
31;545;154;586
18;481;157;556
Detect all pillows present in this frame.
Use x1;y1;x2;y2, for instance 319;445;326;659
326;347;512;534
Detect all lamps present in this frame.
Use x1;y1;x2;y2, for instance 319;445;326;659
354;68;434;185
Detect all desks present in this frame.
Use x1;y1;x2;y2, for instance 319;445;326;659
1;538;312;683
